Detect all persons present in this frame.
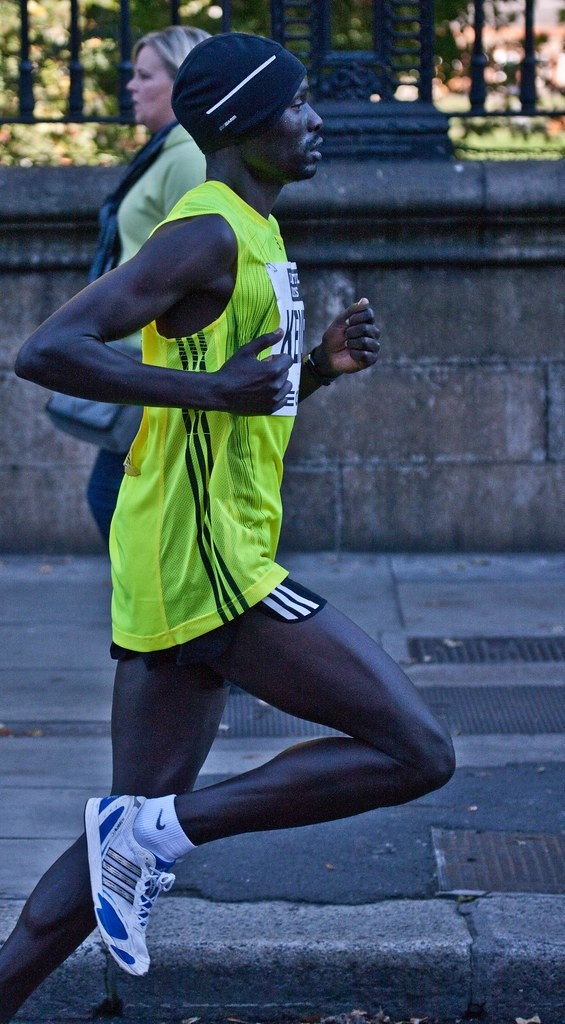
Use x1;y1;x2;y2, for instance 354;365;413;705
1;31;455;1024
88;28;214;556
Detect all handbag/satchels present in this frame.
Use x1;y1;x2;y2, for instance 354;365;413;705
44;339;155;454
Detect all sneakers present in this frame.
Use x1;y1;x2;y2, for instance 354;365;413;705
84;793;178;978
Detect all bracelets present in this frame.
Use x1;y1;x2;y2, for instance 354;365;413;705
303;346;331;386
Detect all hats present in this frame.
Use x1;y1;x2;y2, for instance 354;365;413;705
173;33;307;157
